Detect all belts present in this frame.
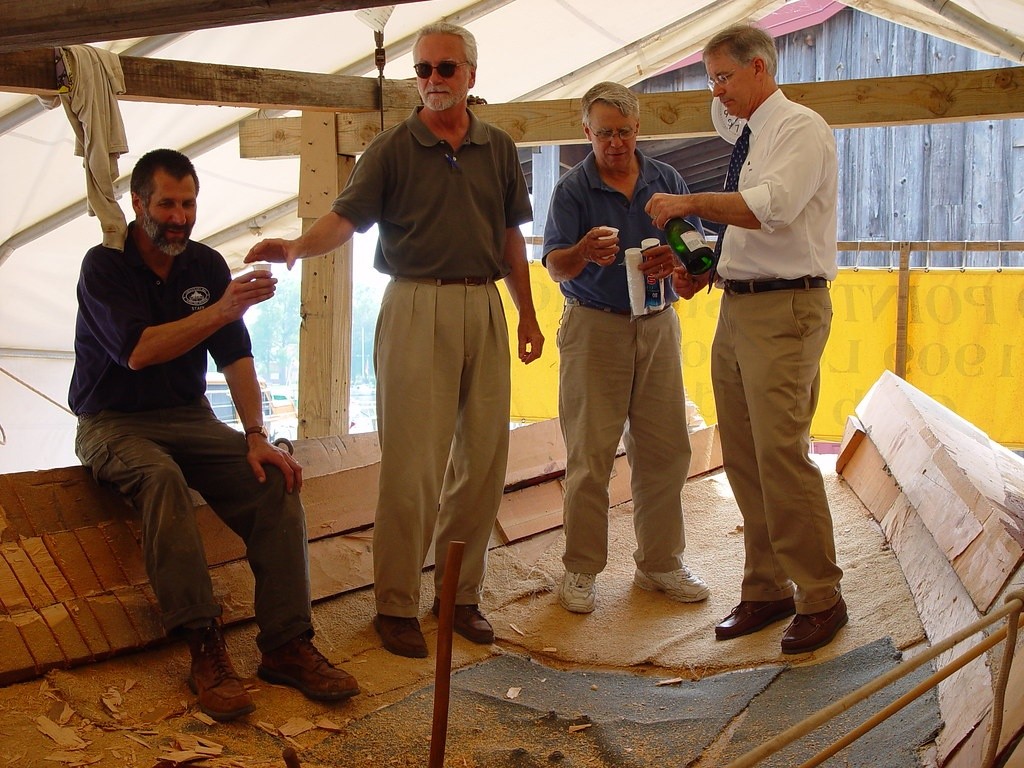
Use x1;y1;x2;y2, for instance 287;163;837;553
79;409;102;420
567;296;671;321
724;276;828;294
398;270;487;286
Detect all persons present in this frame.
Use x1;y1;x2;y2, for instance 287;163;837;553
541;81;710;614
245;22;544;658
644;27;851;656
68;149;360;720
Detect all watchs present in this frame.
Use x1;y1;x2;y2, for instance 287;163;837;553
245;426;268;441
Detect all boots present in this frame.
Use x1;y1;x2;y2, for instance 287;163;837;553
181;626;257;719
257;631;362;698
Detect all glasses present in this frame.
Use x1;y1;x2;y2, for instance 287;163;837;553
413;60;468;79
707;58;755;92
586;121;639;142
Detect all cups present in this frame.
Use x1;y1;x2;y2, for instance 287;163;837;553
253;264;271;281
598;226;619;259
625;237;665;316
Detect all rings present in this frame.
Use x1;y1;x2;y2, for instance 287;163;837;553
651;214;657;220
296;461;299;464
661;264;663;269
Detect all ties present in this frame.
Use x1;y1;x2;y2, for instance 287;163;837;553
707;124;752;295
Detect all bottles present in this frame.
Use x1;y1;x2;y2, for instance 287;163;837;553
661;216;714;275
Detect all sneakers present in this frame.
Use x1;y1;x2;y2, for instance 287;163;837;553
558;567;596;614
374;609;429;658
781;597;848;654
715;596;795;639
636;566;710;602
433;595;495;644
